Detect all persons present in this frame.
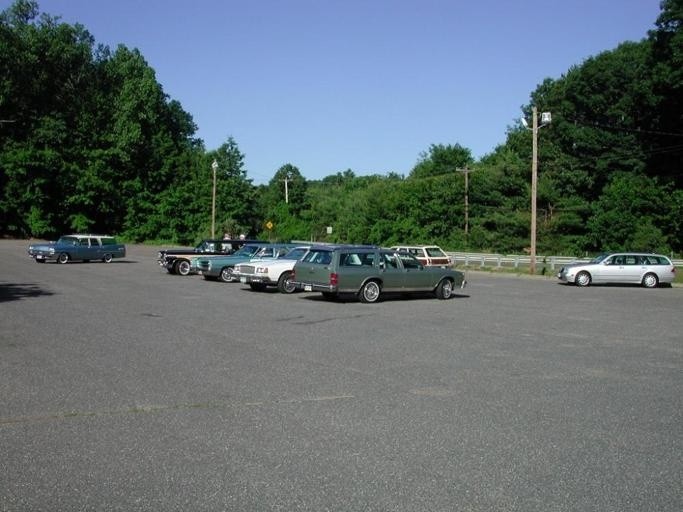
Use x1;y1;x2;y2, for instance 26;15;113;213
219;231;232;252
234;234;246;251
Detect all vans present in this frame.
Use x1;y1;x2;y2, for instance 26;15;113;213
558;251;676;288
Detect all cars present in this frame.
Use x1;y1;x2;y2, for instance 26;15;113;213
27;234;125;264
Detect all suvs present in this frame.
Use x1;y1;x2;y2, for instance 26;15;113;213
230;246;393;294
157;238;270;277
288;244;466;302
390;245;452;271
195;243;307;284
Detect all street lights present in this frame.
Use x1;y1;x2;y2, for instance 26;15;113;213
209;158;218;241
519;106;551;275
